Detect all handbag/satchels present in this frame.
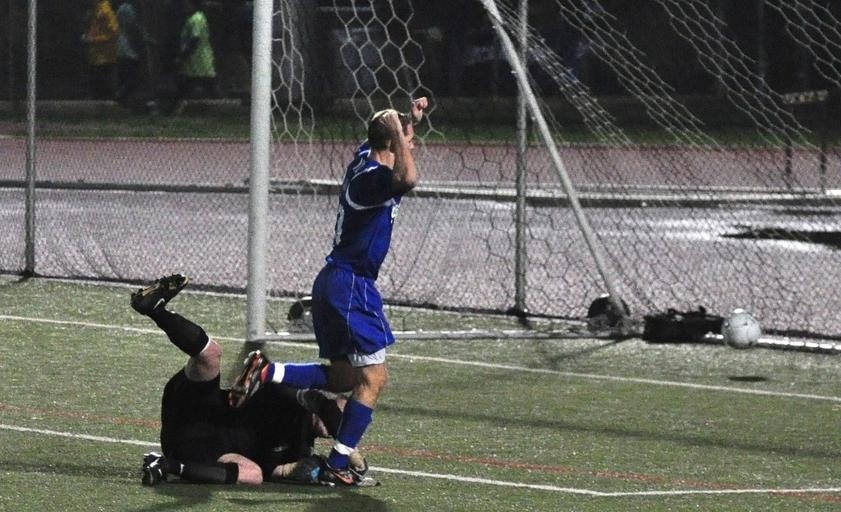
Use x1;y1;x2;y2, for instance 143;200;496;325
643;305;725;343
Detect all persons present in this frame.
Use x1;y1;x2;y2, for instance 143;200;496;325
228;96;429;487
80;0;243;118
130;274;370;486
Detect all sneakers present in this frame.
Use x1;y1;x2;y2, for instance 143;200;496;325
228;349;270;408
141;452;165;487
318;461;380;487
130;273;188;313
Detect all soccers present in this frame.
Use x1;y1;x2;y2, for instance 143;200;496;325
723;307;763;348
